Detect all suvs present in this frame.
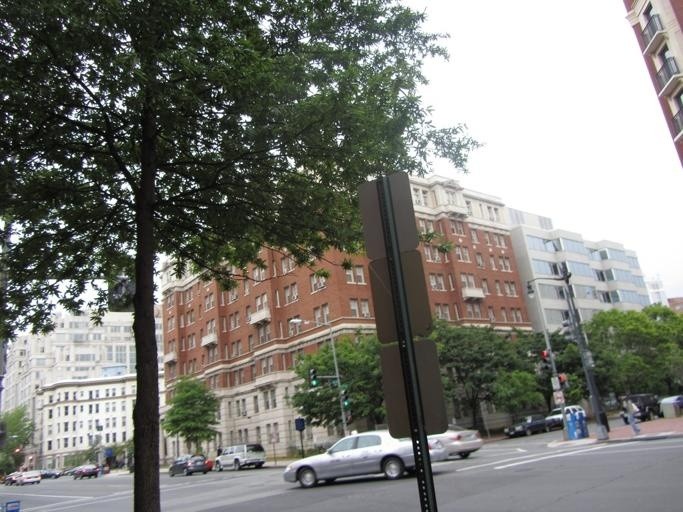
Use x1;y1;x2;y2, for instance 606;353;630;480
545;405;586;433
620;394;664;424
215;444;265;471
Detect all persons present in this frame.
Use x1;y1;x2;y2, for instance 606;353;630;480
576;407;588;438
218;445;222;456
565;408;577;440
622;395;641;434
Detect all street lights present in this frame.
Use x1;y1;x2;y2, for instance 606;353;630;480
527;270;612;431
290;318;348;436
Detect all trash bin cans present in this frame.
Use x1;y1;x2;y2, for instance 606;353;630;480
563;405;589;440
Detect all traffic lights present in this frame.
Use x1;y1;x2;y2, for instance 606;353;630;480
308;368;317;388
342;389;349;408
541;350;552;371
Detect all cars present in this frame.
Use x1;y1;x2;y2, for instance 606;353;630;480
169;454;208;477
63;465;100;480
502;414;547;437
283;424;484;488
5;470;61;486
